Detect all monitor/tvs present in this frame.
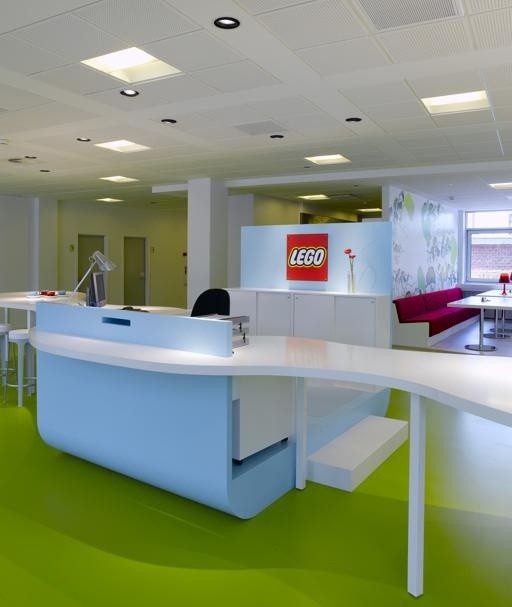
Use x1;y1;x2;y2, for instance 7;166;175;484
86;272;107;307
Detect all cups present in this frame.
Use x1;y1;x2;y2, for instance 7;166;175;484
47;291;55;296
39;290;47;295
58;290;66;295
30;292;37;296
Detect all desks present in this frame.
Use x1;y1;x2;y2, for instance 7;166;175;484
447;290;512;352
0;290;85;394
27;301;512;599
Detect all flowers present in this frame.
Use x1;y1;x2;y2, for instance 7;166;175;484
344;249;357;272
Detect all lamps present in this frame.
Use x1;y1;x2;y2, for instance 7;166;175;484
65;251;118;304
499;274;511;295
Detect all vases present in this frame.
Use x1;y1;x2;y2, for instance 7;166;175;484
347;272;355;293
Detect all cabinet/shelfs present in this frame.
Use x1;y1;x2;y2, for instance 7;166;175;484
221;287;294;337
292;290;391;349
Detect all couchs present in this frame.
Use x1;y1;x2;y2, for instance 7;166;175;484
392;288;486;348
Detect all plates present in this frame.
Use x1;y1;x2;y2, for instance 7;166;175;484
58;295;68;297
27;296;40;298
45;296;57;298
40;295;45;297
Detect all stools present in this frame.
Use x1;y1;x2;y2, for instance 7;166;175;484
8;330;30;407
0;324;13;400
